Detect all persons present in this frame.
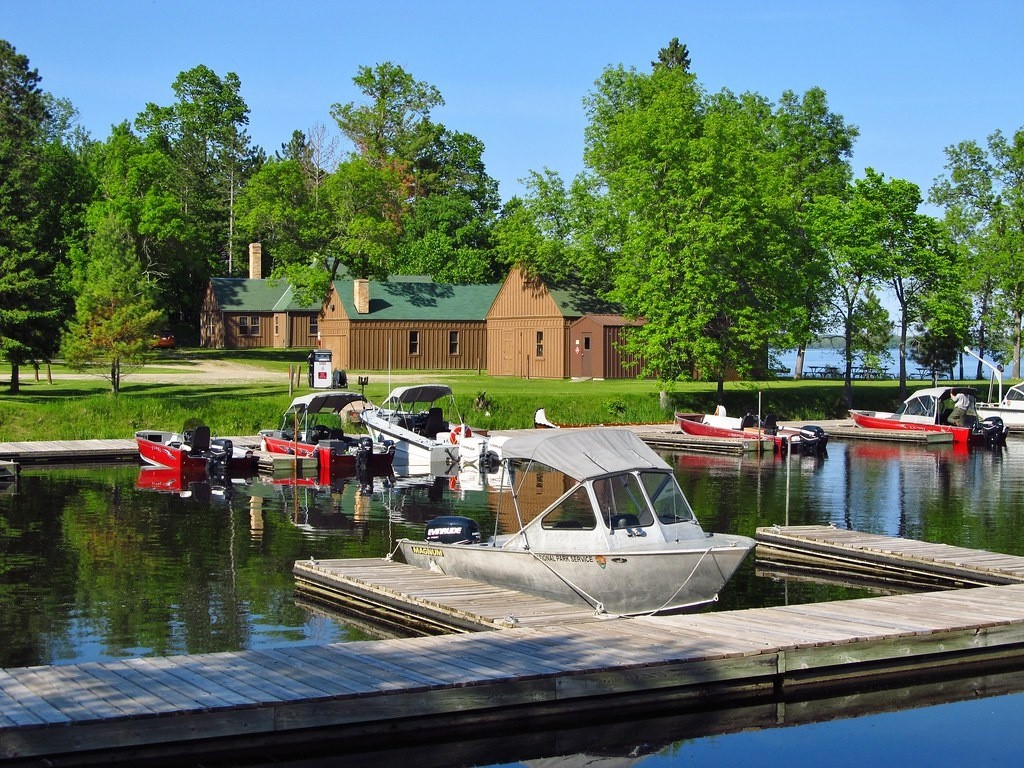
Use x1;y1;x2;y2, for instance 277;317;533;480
947;388;971;427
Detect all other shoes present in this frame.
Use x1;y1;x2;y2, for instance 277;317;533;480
956;419;960;427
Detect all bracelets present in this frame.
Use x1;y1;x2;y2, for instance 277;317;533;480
950;392;952;393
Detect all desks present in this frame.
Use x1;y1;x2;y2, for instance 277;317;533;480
850;366;871;379
915;368;943;380
808;366;839;379
872;367;891;379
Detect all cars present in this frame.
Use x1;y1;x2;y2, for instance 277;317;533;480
150;330;175;349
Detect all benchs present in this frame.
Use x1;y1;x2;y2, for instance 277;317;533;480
909;373;949;379
827;372;846;374
802;371;831;375
843;371;871;374
876;373;894;375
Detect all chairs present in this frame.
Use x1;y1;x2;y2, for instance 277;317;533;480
939;408;954;424
328;429;344;441
758;414;777;426
733;415;753;429
553;520;584;530
609;513;640;528
190;425;209;455
423;408;445;436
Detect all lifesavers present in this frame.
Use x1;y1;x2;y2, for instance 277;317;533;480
448;424;472;445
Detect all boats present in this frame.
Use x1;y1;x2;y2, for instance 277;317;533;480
230;445;260;468
256;391;398;474
974;381;1024;433
672;405;830;454
359;384;479;479
847;386;986;445
135;423;232;477
397;426;761;617
133;465;210;501
